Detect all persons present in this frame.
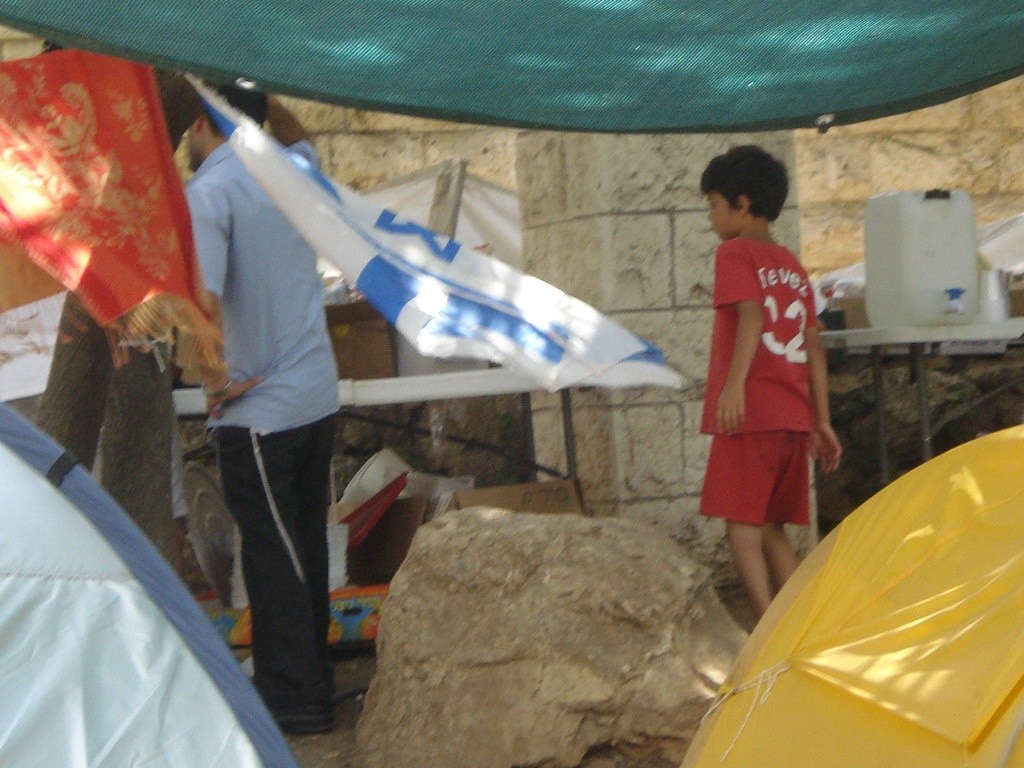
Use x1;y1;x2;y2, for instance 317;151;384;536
187;76;339;735
702;148;843;621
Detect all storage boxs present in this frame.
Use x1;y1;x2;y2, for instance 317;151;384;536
445;480;582;514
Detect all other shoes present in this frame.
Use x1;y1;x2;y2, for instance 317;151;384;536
264;696;336;735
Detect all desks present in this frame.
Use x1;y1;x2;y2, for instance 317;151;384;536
172;360;686;518
819;316;1024;484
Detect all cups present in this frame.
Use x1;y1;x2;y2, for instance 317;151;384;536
980;269;1006;323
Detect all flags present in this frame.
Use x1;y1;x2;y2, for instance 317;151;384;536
2;53;226;388
191;75;691;393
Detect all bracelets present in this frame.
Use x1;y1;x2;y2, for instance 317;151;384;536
205;381;238;396
